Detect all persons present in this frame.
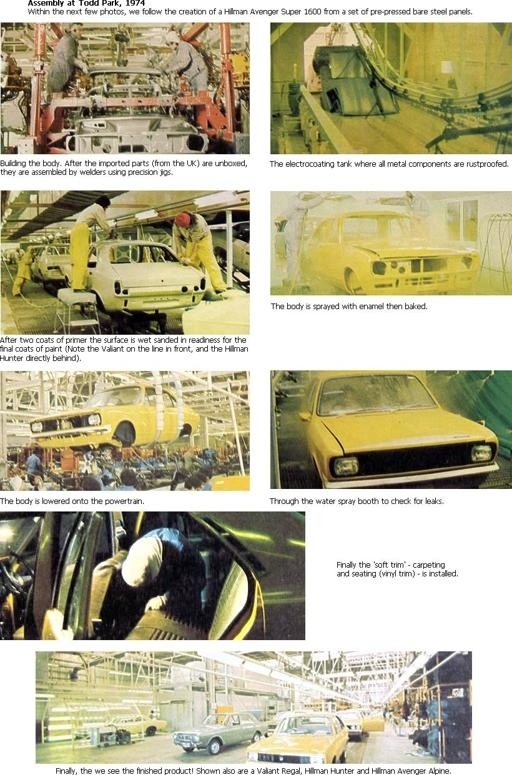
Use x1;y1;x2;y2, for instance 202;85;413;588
280;190;329;287
5;445;235;491
114;527;208;639
46;22;90;103
149;711;155;719
71;194;119;294
367;190;433;220
11;248;36;296
171;210;228;301
158;32;208;95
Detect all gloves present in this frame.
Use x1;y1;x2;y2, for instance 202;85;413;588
144;594;168;614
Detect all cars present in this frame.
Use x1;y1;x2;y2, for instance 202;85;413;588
32;386;202;454
1;511;306;641
2;235;210;313
44;60;213;158
291;210;485;298
302;372;498;489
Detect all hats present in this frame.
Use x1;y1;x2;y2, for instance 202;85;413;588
121;535;163;588
175;213;191;228
167;31;181;44
